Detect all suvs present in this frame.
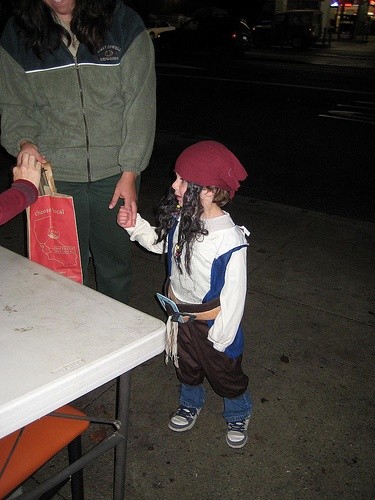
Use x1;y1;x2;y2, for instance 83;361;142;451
155;14;254;58
250;10;322;50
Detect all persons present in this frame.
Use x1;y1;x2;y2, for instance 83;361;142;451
0;0;157;305
117;140;251;449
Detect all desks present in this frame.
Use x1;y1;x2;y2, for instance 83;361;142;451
0;245;169;500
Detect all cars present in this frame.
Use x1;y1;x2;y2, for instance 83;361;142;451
193;7;229;19
144;20;175;39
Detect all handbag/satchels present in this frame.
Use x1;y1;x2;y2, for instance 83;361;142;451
26;161;83;286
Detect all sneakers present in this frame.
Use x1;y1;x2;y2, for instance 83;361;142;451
225;414;252;449
168;404;202;432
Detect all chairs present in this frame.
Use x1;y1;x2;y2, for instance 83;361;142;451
0;404;89;500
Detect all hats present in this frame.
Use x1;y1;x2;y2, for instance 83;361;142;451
174;140;249;206
